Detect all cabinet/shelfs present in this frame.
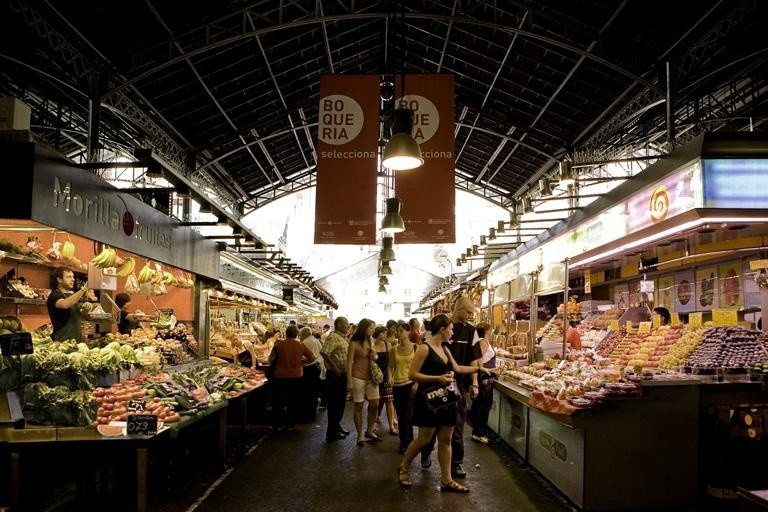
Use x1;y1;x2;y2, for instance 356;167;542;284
487;308;768;512
2;248;288;510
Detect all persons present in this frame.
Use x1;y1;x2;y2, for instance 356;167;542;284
47;267;89;343
115;292;145;336
230;334;259;371
650;308;670;327
166;309;177;335
554;314;581;351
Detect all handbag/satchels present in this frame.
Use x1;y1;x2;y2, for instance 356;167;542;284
368;361;383;384
423;382;462;412
265;359;277;381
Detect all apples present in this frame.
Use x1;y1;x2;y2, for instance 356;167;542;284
685;323;767;371
574;310;600;338
616;324;682;369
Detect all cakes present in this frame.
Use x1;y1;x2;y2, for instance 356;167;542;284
650;186;670;223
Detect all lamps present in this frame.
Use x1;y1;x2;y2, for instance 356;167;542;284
381;261;393;274
380;72;425;172
456;154;667;267
78;146;268;251
380;236;397;261
379;198;407;234
379;276;390;285
313;289;339;310
418;272;471;308
378;285;387;293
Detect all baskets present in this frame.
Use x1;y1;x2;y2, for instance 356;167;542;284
132;313;158;322
86;312;117;323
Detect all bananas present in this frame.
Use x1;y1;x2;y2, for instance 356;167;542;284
136;265;152;283
152;271;193;296
91;249;116;268
63;240;75;264
118;257;134;277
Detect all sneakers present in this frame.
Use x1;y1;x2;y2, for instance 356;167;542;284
264;405;409;455
451;461;466;478
420;451;432;469
471;432;493;444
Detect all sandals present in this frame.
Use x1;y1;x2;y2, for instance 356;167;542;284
441;479;471;493
397;466;413;487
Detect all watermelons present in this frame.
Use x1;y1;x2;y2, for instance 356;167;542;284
97;425;125;437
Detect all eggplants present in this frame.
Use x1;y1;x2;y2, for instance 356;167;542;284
0;318;20;336
150;381;207;415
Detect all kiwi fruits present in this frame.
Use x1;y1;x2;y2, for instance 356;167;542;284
594;308;622;328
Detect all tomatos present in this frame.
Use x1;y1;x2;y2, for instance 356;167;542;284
89;374;179;423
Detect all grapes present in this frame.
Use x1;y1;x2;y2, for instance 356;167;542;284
659;328;704;371
